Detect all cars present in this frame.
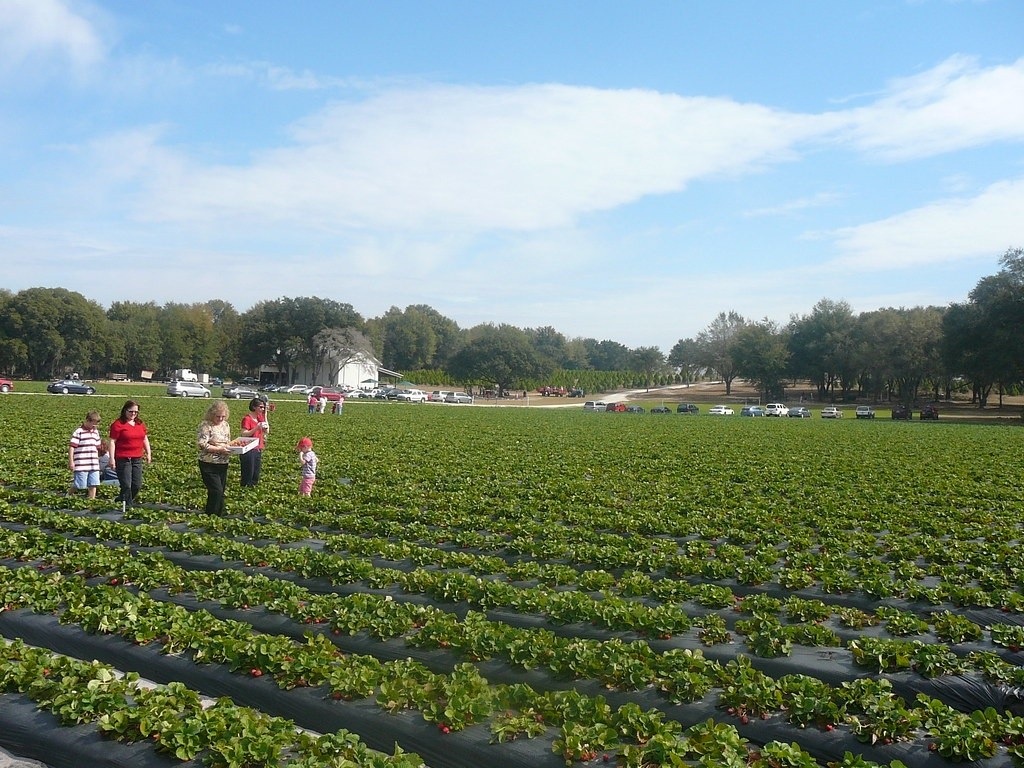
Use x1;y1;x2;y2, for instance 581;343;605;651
166;381;211;398
788;406;812;419
357;388;433;403
300;385;324;396
920;407;938;420
343;389;366;398
539;385;585;398
258;383;308;394
46;379;97;395
741;405;765;417
821;407;844;419
856;406;875;419
627;405;646;413
444;391;473;404
238;377;260;385
0;378;14;393
222;385;261;399
708;405;735;415
213;377;222;386
431;390;450;402
651;407;672;413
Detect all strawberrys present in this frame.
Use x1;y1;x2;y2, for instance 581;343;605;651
0;562;1024;768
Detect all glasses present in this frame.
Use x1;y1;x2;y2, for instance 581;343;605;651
127;409;139;414
257;405;265;409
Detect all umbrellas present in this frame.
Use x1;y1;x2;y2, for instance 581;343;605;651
361;379;381;384
400;381;414;389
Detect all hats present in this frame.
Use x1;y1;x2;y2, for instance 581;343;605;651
296;438;312;449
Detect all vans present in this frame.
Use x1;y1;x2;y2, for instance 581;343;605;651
892;404;913;420
677;403;700;414
607;402;628;412
583;401;608;412
312;388;344;401
764;402;790;418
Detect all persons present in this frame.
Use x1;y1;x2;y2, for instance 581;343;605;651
98;439;120;485
66;411;102;498
331;397;343;415
308;395;327;414
109;400;151;505
297;439;318;497
240;398;269;486
198;401;233;517
262;395;275;411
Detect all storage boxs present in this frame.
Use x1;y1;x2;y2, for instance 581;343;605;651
224;436;259;454
